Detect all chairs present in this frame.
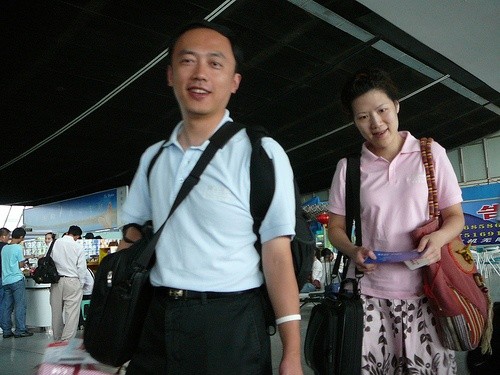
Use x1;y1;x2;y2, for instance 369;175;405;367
298;262;329;310
468;245;500;278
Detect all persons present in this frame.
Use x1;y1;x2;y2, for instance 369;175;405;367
469;302;500;375
34;232;56;259
116;23;303;374
78;265;95;330
1;227;34;338
44;225;87;345
300;247;323;295
61;232;102;239
326;81;466;375
0;227;12;305
321;248;344;285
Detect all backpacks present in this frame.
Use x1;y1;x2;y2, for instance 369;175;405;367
146;124;317;337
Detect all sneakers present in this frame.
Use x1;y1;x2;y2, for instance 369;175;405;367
14;331;33;338
3;332;14;338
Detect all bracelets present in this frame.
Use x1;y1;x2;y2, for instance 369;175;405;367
276;314;302;326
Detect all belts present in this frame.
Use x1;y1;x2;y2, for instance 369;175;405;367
168;286;246;300
60;275;68;277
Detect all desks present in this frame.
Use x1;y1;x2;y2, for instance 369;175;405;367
12;279;53;331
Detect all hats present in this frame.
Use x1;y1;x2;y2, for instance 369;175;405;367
69;226;82;239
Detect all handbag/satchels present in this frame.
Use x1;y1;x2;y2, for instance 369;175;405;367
411;137;494;355
304;278;363;375
83;223;156;367
33;239;60;284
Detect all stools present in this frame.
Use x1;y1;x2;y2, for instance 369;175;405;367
81;299;91;328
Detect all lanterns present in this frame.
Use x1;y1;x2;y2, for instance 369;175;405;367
317;212;330;228
